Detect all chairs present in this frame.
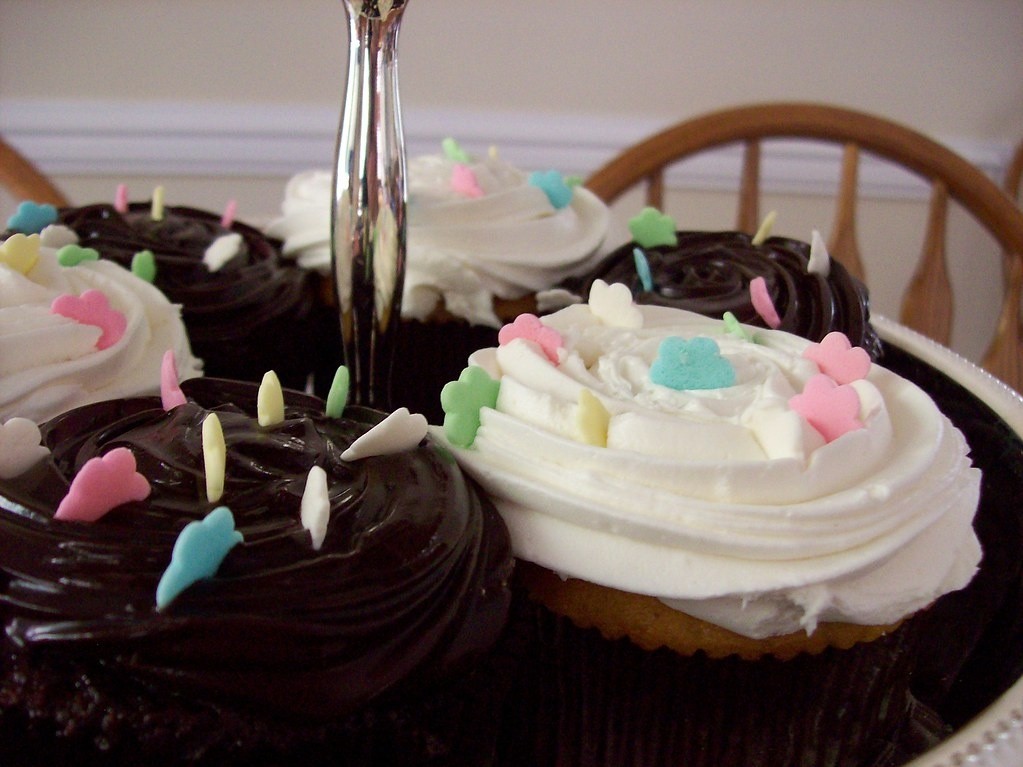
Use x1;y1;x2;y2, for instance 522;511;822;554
584;103;1023;400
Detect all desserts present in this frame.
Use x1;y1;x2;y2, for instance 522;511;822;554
0;131;985;767
